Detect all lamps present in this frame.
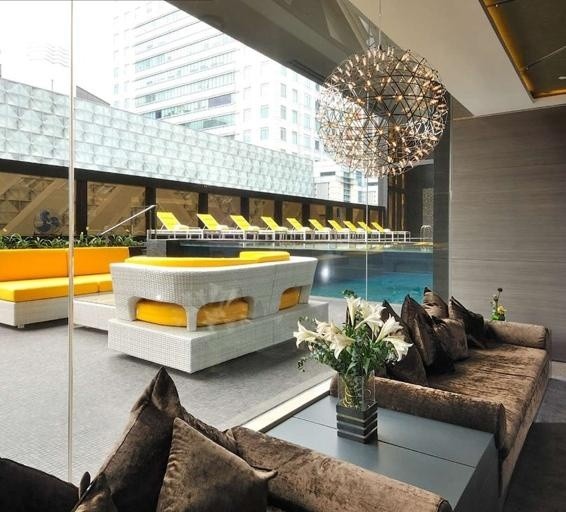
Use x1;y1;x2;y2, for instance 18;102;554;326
313;0;450;179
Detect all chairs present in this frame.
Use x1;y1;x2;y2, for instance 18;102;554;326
155;210;400;242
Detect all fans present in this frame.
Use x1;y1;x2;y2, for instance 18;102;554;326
33;207;65;236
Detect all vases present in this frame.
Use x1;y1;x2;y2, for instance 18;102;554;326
335;370;379;445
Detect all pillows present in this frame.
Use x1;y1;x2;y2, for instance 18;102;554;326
380;286;489;389
69;365;278;512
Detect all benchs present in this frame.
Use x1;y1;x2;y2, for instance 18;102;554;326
106;250;330;376
330;319;551;502
0;244;130;329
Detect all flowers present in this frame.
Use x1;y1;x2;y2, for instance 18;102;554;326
292;288;415;410
490;286;507;322
